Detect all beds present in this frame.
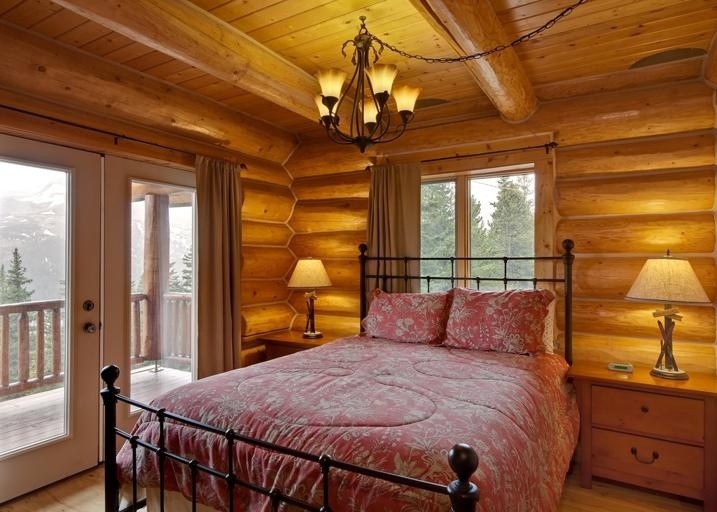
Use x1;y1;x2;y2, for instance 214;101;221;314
94;238;581;509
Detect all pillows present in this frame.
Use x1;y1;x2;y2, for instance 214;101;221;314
360;281;560;361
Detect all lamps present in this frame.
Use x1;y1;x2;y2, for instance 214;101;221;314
284;250;332;339
312;0;593;153
625;245;713;382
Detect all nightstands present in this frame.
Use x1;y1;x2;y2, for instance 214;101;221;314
250;328;343;358
563;353;717;511
